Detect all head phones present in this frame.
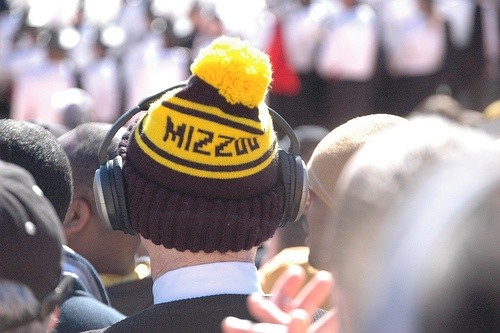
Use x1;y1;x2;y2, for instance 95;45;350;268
94;80;309;236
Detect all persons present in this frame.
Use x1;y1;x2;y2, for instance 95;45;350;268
2;0;500;137
0;36;500;333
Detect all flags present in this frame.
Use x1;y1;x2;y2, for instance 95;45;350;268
261;12;302;95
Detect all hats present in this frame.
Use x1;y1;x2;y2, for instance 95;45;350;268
122;35;285;253
307;113;408;212
0;160;78;333
